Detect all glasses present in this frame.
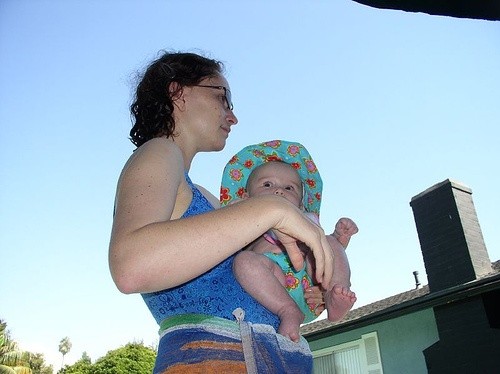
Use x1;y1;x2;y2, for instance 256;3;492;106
192;84;233;111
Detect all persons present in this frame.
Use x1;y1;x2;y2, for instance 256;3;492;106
105;47;334;374
217;136;359;335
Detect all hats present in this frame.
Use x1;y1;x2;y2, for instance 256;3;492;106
219;139;323;220
